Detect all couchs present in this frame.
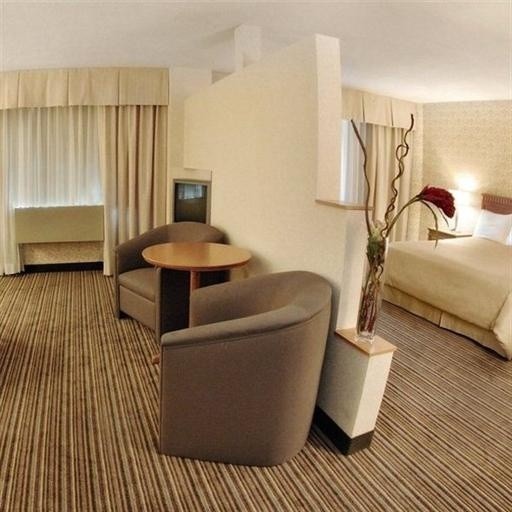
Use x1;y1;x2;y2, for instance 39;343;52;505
158;269;333;469
110;222;224;342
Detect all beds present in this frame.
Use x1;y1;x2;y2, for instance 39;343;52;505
382;194;512;362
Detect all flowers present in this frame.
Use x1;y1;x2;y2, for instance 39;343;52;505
349;113;458;330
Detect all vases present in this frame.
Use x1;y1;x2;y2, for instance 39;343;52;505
356;243;385;341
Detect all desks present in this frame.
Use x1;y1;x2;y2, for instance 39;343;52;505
142;242;251;361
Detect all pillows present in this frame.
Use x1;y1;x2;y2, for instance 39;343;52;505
474;211;511;244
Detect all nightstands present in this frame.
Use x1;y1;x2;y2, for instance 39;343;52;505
427;227;473;243
